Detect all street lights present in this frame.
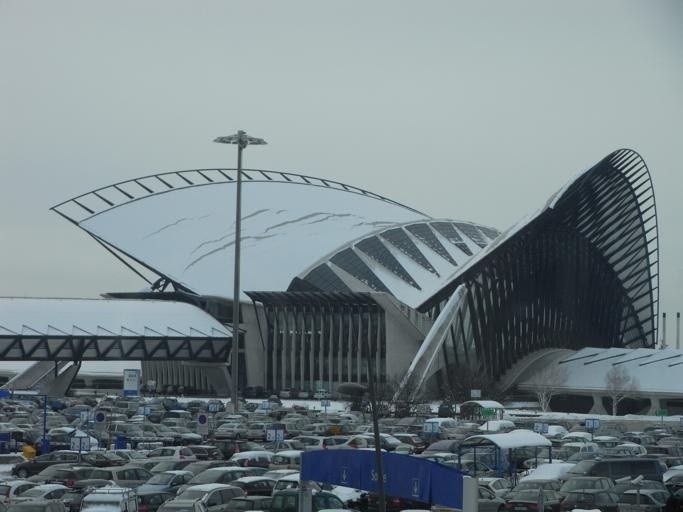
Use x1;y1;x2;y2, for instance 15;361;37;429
211;127;268;410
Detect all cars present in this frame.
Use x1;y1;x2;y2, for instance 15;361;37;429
0;388;682;511
